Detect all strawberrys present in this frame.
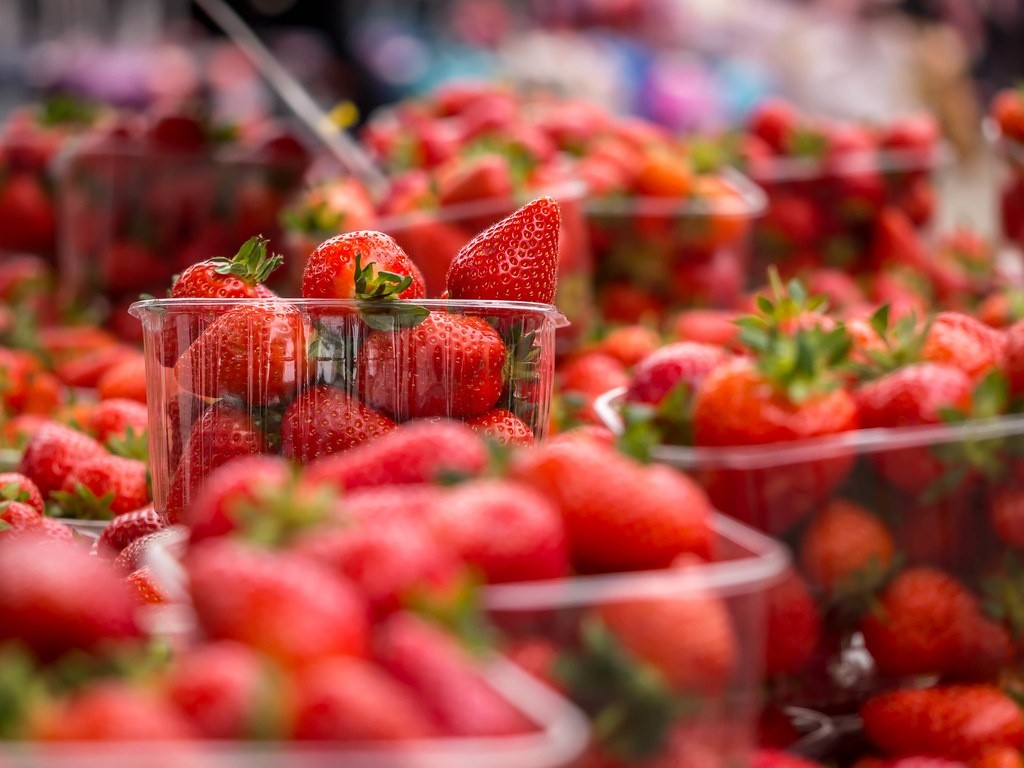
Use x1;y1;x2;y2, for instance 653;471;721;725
0;70;1024;768
